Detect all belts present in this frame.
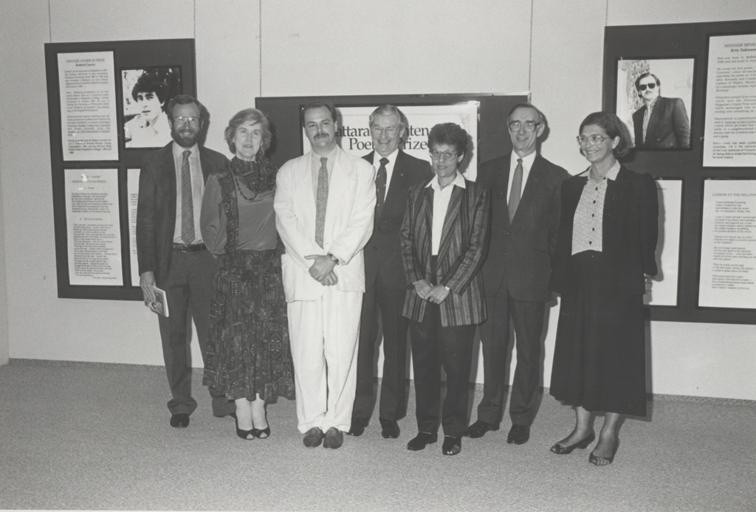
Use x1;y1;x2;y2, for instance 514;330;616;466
172;243;204;253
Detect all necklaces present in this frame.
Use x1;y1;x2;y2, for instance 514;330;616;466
232;166;261;201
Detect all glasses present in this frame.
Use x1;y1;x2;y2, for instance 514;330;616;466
175;116;201;124
639;83;656;90
576;134;610;145
509;121;540;132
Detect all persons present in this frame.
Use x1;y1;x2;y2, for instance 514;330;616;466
200;106;296;445
549;111;664;467
460;101;574;447
273;99;377;451
398;122;493;457
631;73;691;148
348;105;432;441
122;74;174;147
134;93;233;432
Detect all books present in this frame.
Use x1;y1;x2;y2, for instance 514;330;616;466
140;282;171;319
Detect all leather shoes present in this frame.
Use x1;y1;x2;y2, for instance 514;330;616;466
171;415;189;428
253;420;270;439
588;437;619;464
441;437;460;455
378;417;399;440
303;429;321;447
506;420;529;444
235;416;255;441
324;427;343;450
551;430;594;454
345;421;365;437
465;420;499;437
406;431;438;451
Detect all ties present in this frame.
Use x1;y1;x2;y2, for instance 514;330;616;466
315;157;329;249
182;151;195;244
508;158;523;225
374;158;389;230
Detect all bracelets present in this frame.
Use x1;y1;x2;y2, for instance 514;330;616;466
443;285;452;292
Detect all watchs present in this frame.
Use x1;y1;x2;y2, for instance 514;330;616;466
327;251;340;265
